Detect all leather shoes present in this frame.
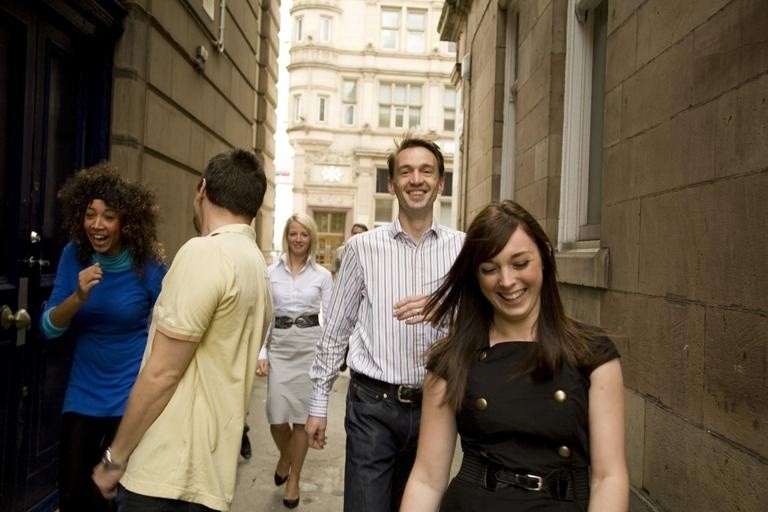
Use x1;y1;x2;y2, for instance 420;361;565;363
284;498;299;508
275;472;288;485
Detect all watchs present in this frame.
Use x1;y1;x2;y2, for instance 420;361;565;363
103;447;127;472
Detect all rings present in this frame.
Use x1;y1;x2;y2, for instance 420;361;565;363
256;368;261;373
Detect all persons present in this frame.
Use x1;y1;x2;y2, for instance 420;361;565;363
303;132;468;510
257;211;347;508
90;146;273;512
399;200;629;511
416;307;420;315
38;163;172;512
192;212;251;461
330;221;369;373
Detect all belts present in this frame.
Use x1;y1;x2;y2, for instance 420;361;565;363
461;456;591;502
274;315;319;328
355;373;420;404
